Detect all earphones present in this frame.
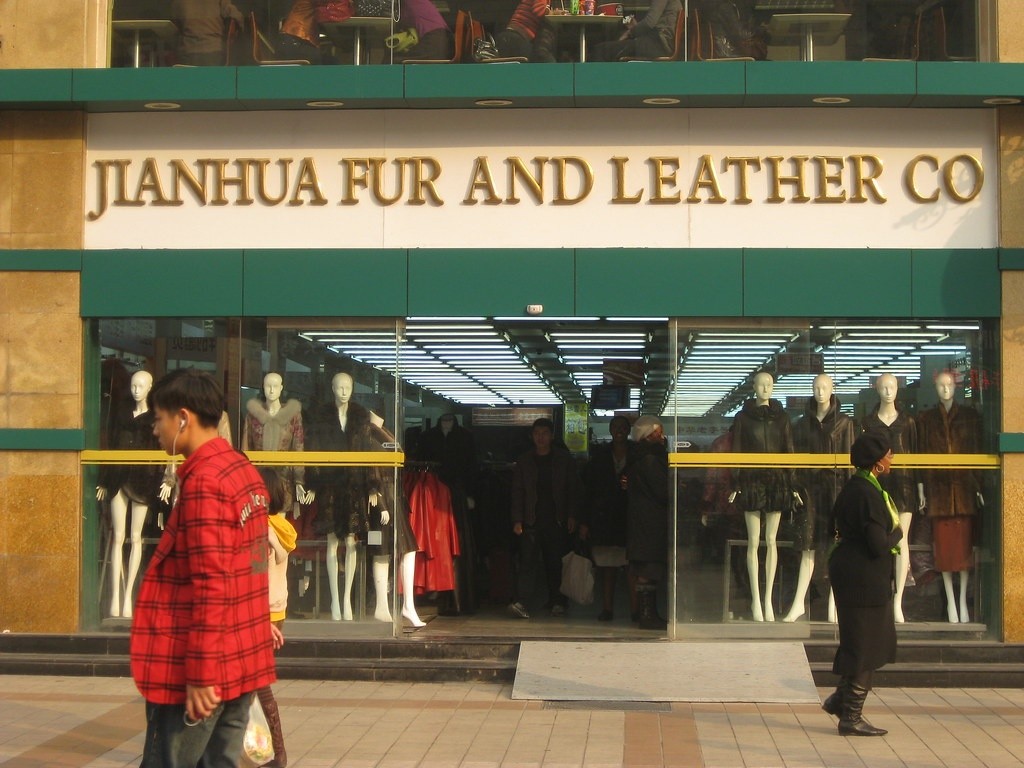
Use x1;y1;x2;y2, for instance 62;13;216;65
180;419;186;429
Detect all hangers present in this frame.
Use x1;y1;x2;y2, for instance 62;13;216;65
400;459;435;477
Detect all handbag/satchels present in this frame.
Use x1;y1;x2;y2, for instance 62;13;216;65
558;535;595;605
823;516;843;571
240;690;275;768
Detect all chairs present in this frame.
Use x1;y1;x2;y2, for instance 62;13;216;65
861;7;924;60
401;10;466;63
171;16;238;67
619;4;684;63
932;5;976;61
691;6;755;61
467;11;529;62
249;10;311;65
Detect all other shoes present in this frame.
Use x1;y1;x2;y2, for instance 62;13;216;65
598;610;614;621
544;605;569;617
508;602;530;618
630;611;638;622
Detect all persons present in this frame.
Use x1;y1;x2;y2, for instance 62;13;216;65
94;370;159;618
510;419;576;619
793;373;985;623
303;372;381;621
131;367;298;768
727;373;805;620
578;415;642;623
366;411;427;628
625;414;668;629
158;409;232;509
167;0;977;66
425;413;473;486
240;372;307;520
821;433;906;737
700;425;745;566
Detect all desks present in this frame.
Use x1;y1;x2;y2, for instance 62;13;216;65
112;18;179;67
765;12;852;60
318;14;396;57
542;14;624;63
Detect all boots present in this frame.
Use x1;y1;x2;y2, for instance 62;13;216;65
261;700;287;768
822;674;848;719
838;682;888;736
635;579;667;630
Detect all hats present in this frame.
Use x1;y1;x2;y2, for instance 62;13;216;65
632;415;662;442
851;430;891;467
